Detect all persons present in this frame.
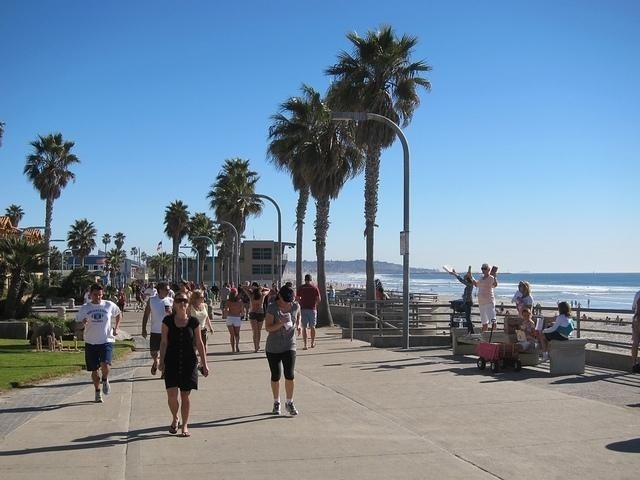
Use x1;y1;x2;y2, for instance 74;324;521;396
449;269;475;335
75;284;122;403
632;290;640;373
103;274;336;380
467;264;497;332
503;281;574;363
158;292;209;438
265;285;302;415
375;279;391;300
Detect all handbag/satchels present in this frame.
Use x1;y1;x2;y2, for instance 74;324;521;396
450;310;469;327
450;299;469;312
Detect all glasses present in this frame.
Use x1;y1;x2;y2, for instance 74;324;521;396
481;268;487;271
175;298;188;303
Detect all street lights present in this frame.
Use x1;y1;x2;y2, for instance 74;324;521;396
326;112;415;353
15;225;86;270
172;234;218;290
210;220;241;290
235;191;283;311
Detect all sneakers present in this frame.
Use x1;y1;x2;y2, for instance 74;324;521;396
151;363;157;375
94;388;103;403
161;371;164;381
102;377;110;394
272;401;281;414
285;401;298;415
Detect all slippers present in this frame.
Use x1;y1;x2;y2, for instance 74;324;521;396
182;431;191;437
169;421;180;434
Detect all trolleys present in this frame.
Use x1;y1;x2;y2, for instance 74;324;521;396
474;318;521;372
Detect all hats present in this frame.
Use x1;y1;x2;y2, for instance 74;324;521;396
280;286;296;302
231;288;238;294
